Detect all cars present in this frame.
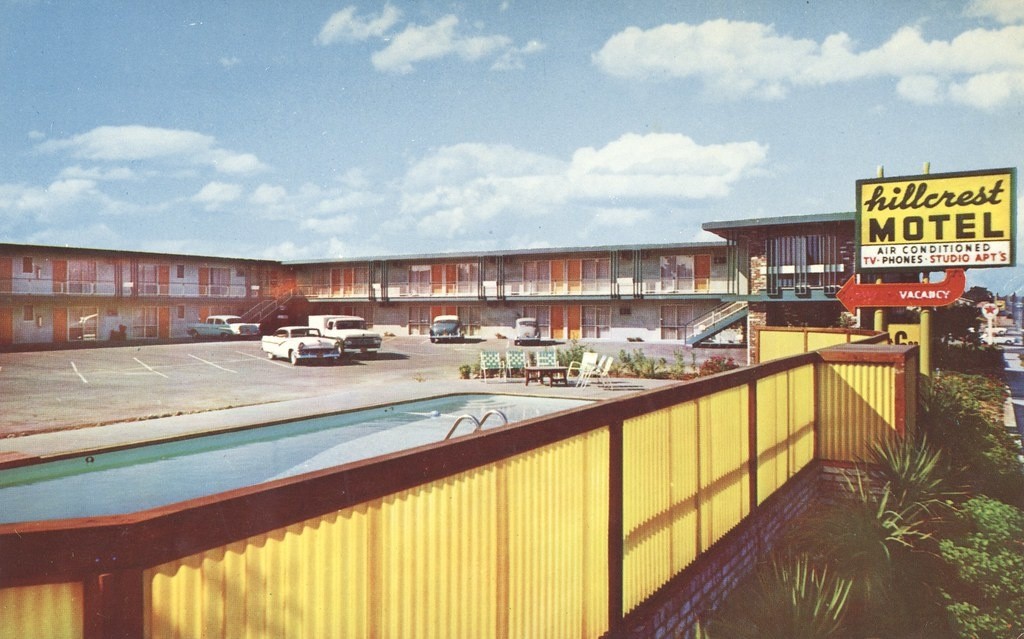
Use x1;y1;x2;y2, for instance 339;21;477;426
261;326;342;366
970;325;1018;344
428;315;465;343
186;314;262;339
514;317;542;345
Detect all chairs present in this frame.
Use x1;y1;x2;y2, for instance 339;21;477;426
480;350;504;379
568;352;615;391
506;350;526;378
535;347;561;384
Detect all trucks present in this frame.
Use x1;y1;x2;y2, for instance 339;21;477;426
309;315;381;354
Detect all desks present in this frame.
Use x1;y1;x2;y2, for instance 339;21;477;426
525;366;569;388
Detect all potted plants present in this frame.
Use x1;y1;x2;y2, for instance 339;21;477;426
459;364;471;379
